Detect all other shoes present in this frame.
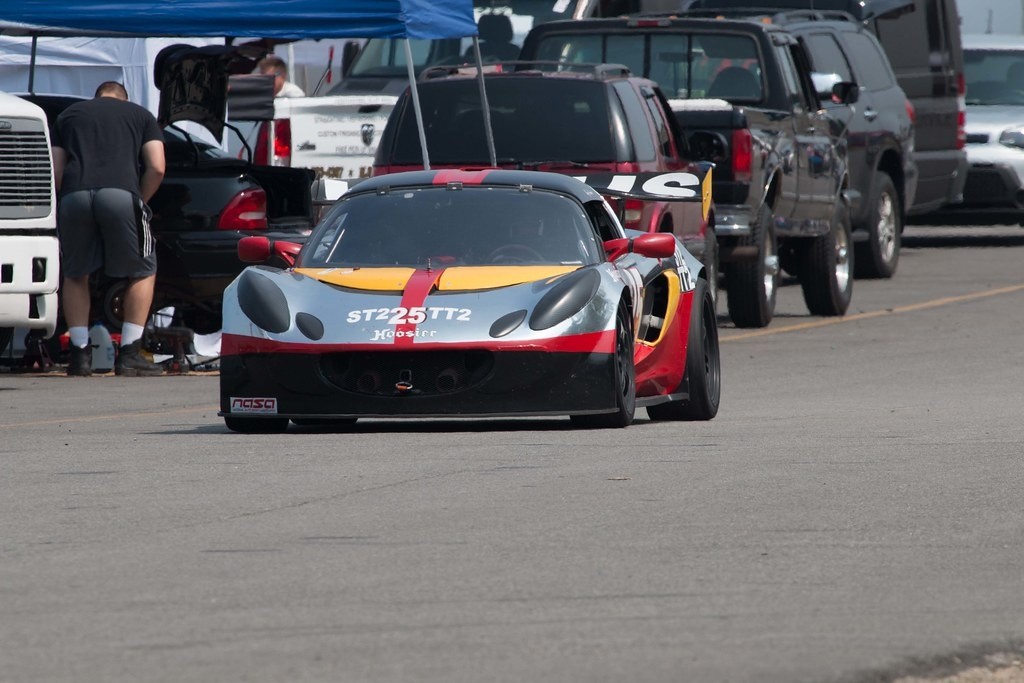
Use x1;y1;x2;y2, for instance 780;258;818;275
115;338;163;376
66;336;99;376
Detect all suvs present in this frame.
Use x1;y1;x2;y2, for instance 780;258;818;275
372;57;720;315
565;9;917;283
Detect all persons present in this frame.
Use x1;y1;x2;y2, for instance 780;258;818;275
49;82;165;376
260;56;305;99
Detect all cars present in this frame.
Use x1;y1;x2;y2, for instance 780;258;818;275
23;95;320;363
959;34;1023;229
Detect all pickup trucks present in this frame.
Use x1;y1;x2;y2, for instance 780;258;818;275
515;19;861;329
226;95;401;195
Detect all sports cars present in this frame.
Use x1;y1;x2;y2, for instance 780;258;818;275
219;162;720;433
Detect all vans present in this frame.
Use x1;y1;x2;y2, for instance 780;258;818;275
326;1;969;237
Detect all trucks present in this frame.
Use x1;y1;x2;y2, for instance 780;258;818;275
0;87;60;365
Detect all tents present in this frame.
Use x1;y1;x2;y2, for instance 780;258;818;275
0;0;498;172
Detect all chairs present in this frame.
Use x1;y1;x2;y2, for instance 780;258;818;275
706;66;761;99
465;15;520;60
